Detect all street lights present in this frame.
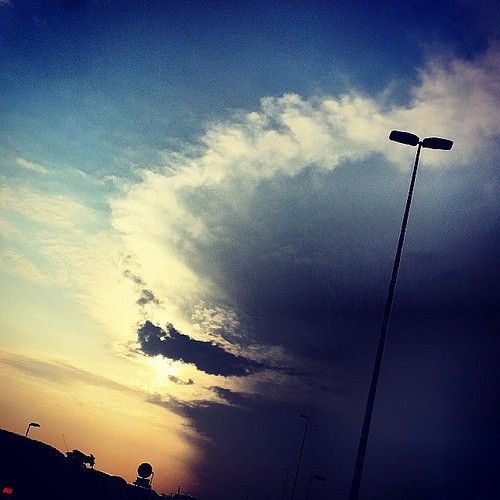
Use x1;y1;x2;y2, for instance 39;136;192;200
346;127;453;499
245;414;328;500
